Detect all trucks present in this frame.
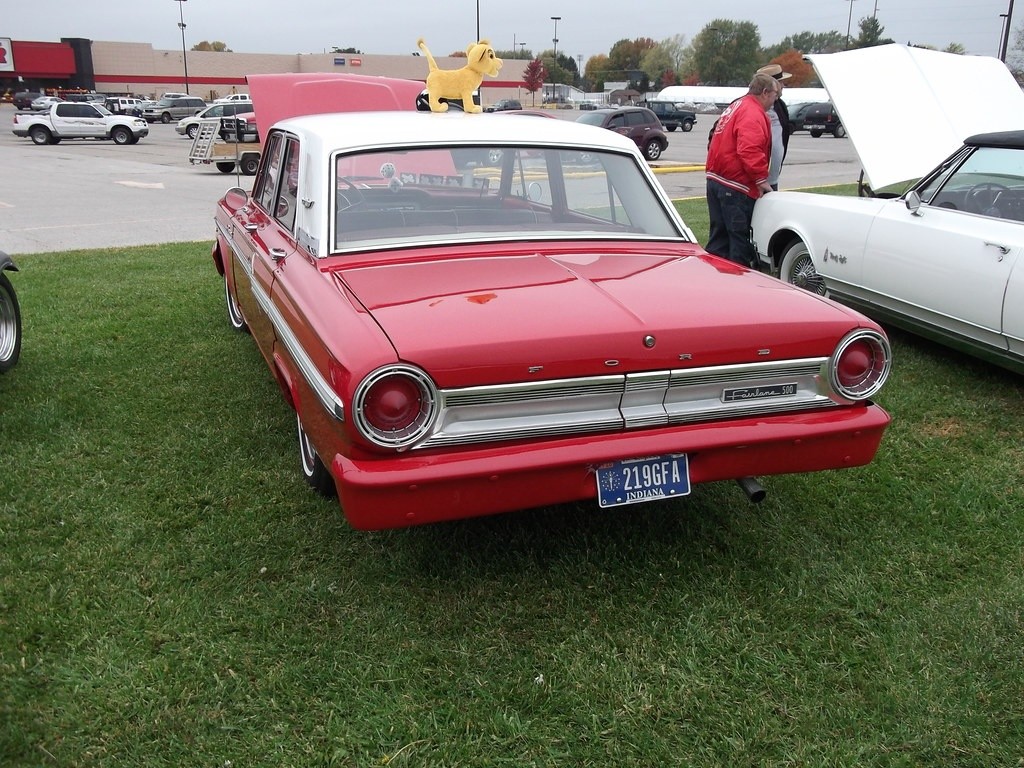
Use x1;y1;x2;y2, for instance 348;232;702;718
189;124;262;175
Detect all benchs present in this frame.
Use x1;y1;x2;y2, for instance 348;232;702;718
335;207;537;228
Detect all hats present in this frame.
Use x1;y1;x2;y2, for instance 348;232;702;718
756;64;793;80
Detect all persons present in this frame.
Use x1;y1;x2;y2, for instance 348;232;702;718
707;64;793;194
705;74;780;268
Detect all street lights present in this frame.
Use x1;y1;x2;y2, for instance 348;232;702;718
577;54;583;77
520;42;526;56
998;15;1008;60
551;17;561;99
175;0;189;95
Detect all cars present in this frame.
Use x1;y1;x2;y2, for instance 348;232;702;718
543;97;619;110
748;45;1024;376
448;101;562;167
211;73;892;530
560;106;670;165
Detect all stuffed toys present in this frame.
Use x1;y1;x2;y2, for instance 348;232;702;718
418;39;503;114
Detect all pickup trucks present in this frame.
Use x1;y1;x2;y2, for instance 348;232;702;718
13;102;149;145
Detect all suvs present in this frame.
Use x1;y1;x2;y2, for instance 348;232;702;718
785;102;847;138
634;100;697;132
0;89;257;138
485;99;523;113
